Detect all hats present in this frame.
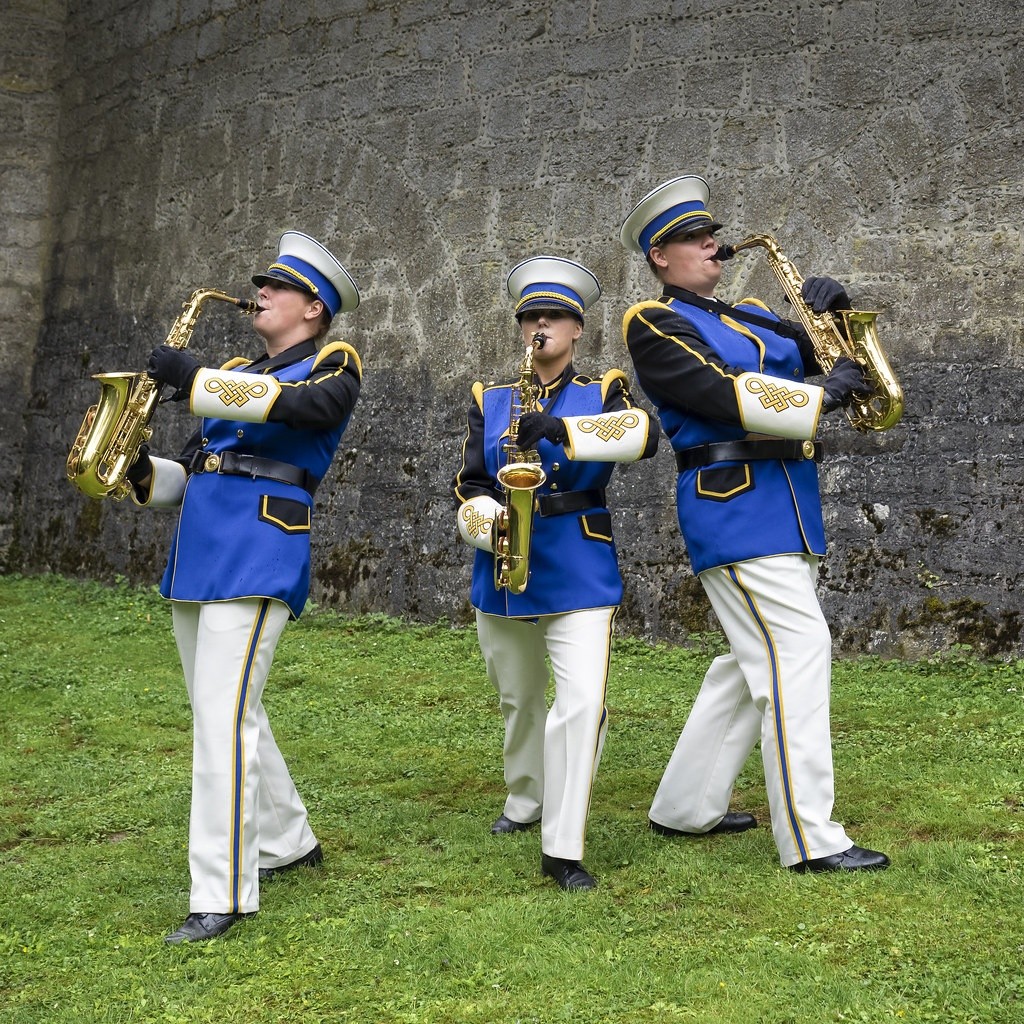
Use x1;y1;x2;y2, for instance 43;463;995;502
619;174;724;259
506;256;602;327
250;231;360;322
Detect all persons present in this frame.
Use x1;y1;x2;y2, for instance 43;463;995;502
620;174;892;874
127;230;362;947
448;255;662;892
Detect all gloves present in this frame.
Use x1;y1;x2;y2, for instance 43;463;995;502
491;518;506;553
124;438;152;484
515;410;565;452
782;277;851;341
820;357;874;417
147;346;201;393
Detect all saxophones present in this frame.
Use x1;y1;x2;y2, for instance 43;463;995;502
65;286;268;505
487;331;548;597
713;234;904;436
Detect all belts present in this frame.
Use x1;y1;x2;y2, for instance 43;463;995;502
188;449;322;499
489;485;607;517
674;440;825;473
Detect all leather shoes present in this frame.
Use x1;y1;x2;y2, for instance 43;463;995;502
789;846;891;875
257;845;324;884
540;853;597;894
492;812;541;835
164;913;254;952
649;813;758;837
